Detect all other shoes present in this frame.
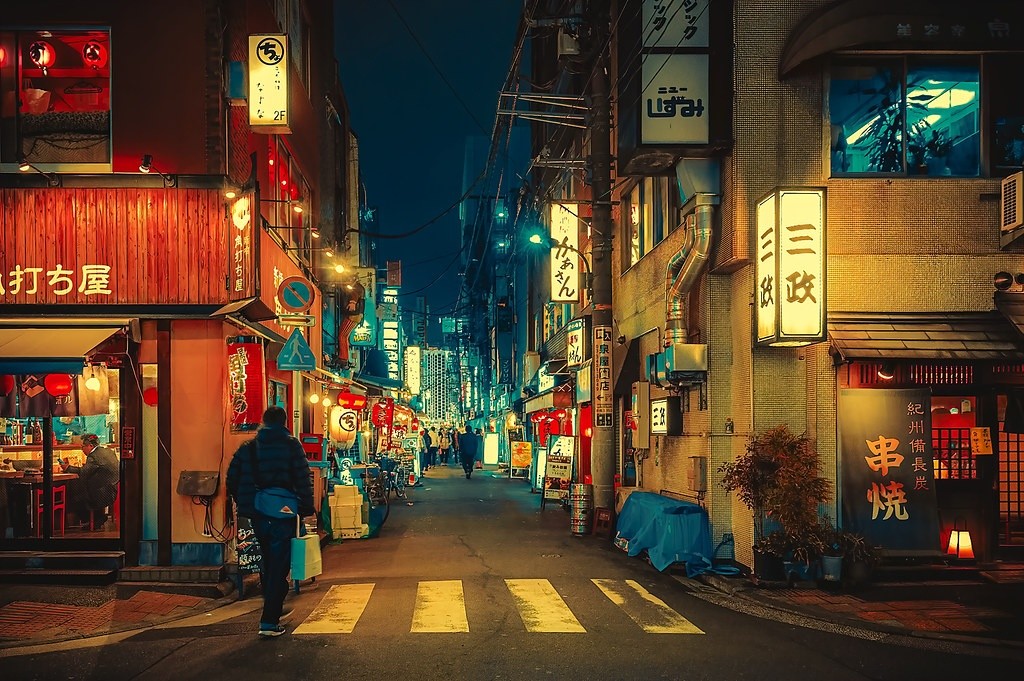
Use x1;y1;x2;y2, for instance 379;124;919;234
422;462;483;479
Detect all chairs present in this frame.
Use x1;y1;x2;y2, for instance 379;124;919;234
90;480;120;532
33;485;65;538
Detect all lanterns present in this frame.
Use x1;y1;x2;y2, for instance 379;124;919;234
143;387;158;407
412;418;418;430
0;375;14;397
337;392;367;410
371;402;388;427
44;373;73;404
538;416;560;447
328;405;357;443
530;409;566;424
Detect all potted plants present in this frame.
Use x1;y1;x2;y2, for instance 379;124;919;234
925;128;964;177
715;425;879;582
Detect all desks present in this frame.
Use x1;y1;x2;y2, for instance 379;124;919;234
0;473;80;538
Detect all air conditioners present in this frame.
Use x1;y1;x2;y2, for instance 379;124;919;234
1001;170;1024;231
557;26;580;62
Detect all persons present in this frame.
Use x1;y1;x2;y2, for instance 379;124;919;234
422;427;483;472
459;425;478;480
60;433;120;530
225;406;316;636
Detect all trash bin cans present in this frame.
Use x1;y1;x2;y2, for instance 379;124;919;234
570;484;594;537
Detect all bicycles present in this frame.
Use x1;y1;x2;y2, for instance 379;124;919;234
358;472;391;538
367;451;408;500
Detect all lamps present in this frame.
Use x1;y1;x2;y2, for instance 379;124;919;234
85;368;100;391
993;272;1024;293
268;225;320;238
305;265;353;289
287;247;335;258
16;154;59;186
260;198;303;213
877;361;896;378
138;155;175;188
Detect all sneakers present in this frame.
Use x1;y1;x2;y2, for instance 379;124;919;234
279;607;295;620
258;624;286;636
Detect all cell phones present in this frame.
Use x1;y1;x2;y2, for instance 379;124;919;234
58;458;65;464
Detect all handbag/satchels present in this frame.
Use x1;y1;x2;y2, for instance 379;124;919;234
254;487;300;520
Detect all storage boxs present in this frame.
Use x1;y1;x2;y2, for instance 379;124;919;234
328;484;370;539
